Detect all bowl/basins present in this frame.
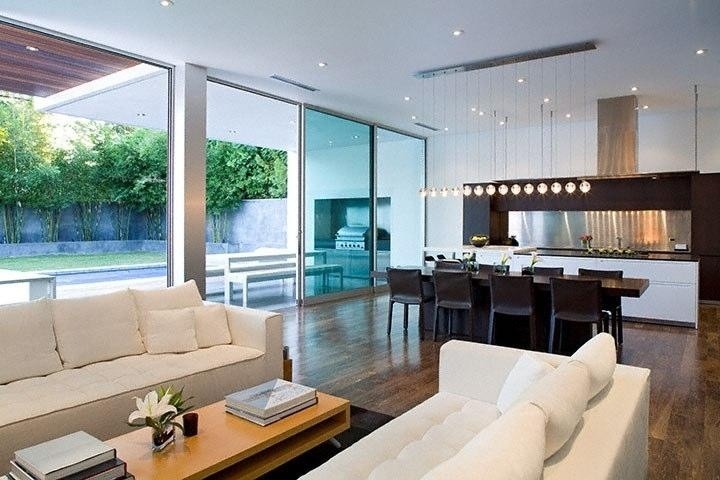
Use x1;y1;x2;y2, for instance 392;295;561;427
469;234;490;247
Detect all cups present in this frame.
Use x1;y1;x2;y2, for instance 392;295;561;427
183;413;198;437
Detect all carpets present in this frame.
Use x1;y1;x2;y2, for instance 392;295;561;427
252;398;396;480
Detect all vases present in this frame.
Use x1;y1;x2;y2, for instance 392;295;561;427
148;421;176;451
467;265;478;274
523;267;534;275
581;240;590;254
494;265;509;275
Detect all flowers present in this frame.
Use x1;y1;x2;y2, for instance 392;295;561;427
580;236;592;241
530;252;542;268
499;252;510;269
119;383;195;431
463;250;478;267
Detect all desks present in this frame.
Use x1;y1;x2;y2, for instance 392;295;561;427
0;270;57;301
390;262;649;357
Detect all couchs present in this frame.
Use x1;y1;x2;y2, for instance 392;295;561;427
0;279;285;471
298;339;653;480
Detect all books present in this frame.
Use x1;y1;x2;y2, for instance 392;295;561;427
4;470;135;479
13;429;116;479
224;396;319;427
7;457;127;480
224;379;317;419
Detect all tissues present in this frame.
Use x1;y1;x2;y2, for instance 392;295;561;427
675;243;687;250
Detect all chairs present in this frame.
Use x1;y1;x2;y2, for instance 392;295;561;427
434;260;466;268
577;267;625;348
385;267;444;339
469;263;508;274
521;265;562;276
548;276;603;354
428;269;483;342
486;272;538;352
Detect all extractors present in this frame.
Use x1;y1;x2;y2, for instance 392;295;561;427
577;96;700;181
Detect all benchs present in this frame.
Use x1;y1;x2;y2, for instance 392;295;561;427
204;248;297;288
223;250;343;309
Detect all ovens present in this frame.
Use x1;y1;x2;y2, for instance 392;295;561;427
335;225;389;251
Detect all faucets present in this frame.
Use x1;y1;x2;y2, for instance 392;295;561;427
616;237;622;249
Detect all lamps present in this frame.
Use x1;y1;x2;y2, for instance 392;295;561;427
565;53;578;194
473;71;484;196
499;65;508;194
486;68;495;195
426;75;437;197
524;61;534;195
461;72;472;196
580;52;590;194
551;55;561;192
439;77;449;196
511;61;521;194
416;76;425;197
536;57;547;194
451;71;459;196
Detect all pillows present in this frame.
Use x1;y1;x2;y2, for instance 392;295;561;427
418;404;546;480
566;331;617;398
125;279;202;343
142;309;198;354
495;353;555;413
529;360;589;459
49;289;145;369
0;296;63;384
192;303;232;349
